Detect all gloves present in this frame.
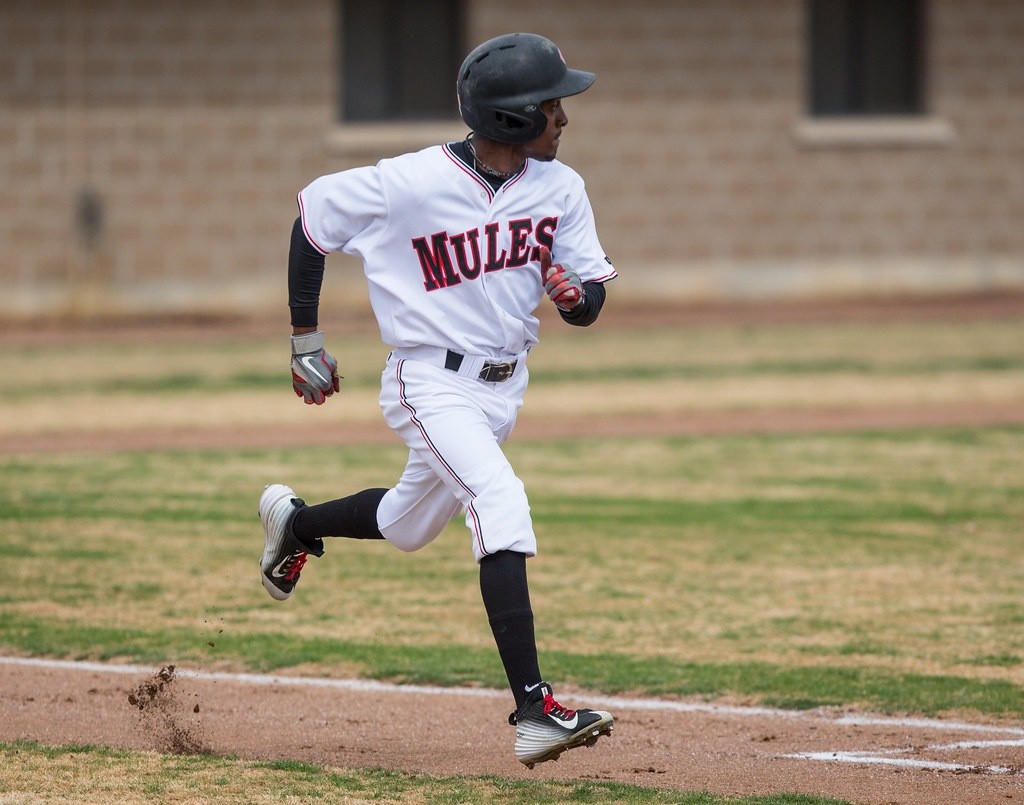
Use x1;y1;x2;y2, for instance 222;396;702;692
540;247;586;312
290;330;340;405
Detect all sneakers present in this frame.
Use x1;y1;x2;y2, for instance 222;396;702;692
258;483;325;601
508;682;614;770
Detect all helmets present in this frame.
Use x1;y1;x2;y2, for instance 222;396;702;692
457;32;597;144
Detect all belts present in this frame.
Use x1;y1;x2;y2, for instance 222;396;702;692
445;350;518;382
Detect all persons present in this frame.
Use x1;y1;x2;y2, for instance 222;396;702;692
258;30;619;769
